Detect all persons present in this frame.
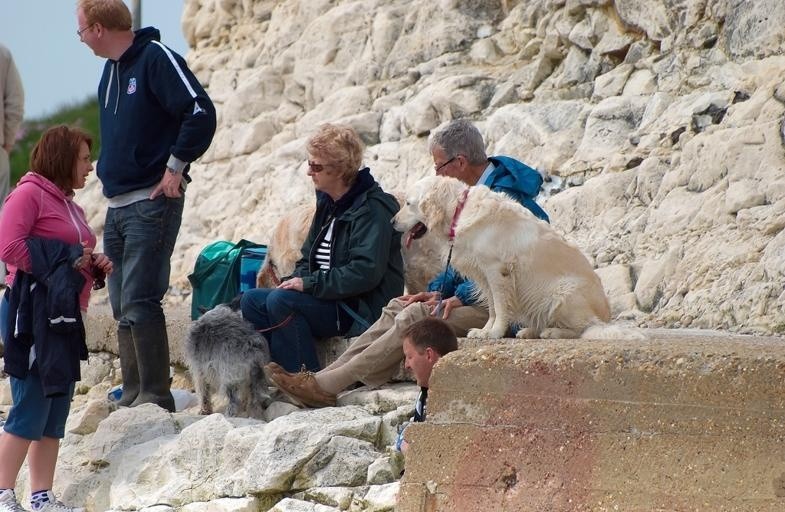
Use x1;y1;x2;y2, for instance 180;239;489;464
1;40;26;289
396;318;457;459
240;125;403;373
0;126;114;512
76;0;216;413
264;119;550;409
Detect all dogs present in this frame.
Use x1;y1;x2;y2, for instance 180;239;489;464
389;174;613;340
184;293;271;416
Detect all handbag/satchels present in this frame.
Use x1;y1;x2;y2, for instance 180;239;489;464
186;238;258;321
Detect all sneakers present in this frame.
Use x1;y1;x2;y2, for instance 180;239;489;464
30;489;87;512
263;361;316;409
268;370;338;409
0;488;31;512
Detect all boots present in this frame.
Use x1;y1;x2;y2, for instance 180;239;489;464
129;321;176;414
115;325;140;406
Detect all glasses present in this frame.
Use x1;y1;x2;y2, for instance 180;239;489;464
79;254;107;291
77;21;98;37
434;157;456;172
308;160;334;173
